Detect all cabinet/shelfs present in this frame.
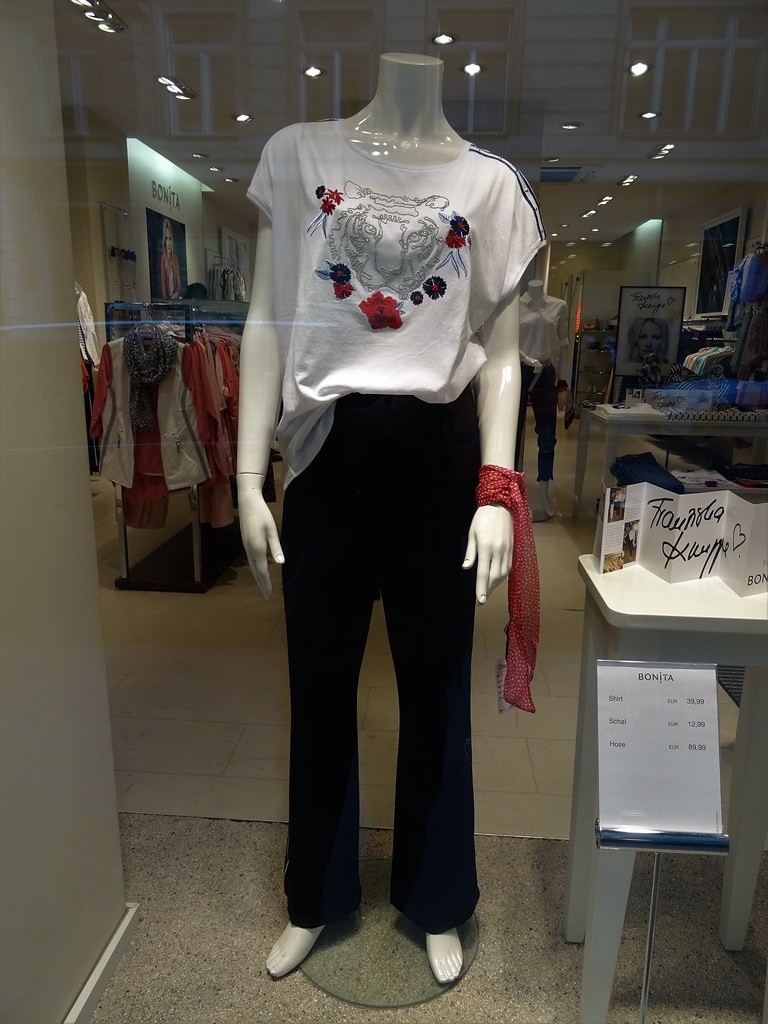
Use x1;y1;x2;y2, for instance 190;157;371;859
573;328;615;419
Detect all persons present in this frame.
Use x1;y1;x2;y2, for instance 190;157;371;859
514;279;570;517
628;318;669;363
160;219;181;299
235;51;547;985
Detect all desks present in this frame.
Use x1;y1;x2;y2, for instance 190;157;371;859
563;551;768;1023
569;402;768;553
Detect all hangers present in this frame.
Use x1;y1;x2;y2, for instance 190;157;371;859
211;255;240;278
130;308;226;332
689;336;738;364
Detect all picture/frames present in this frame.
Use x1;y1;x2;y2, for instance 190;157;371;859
222;227;251;297
691;206;748;319
612;286;687;378
